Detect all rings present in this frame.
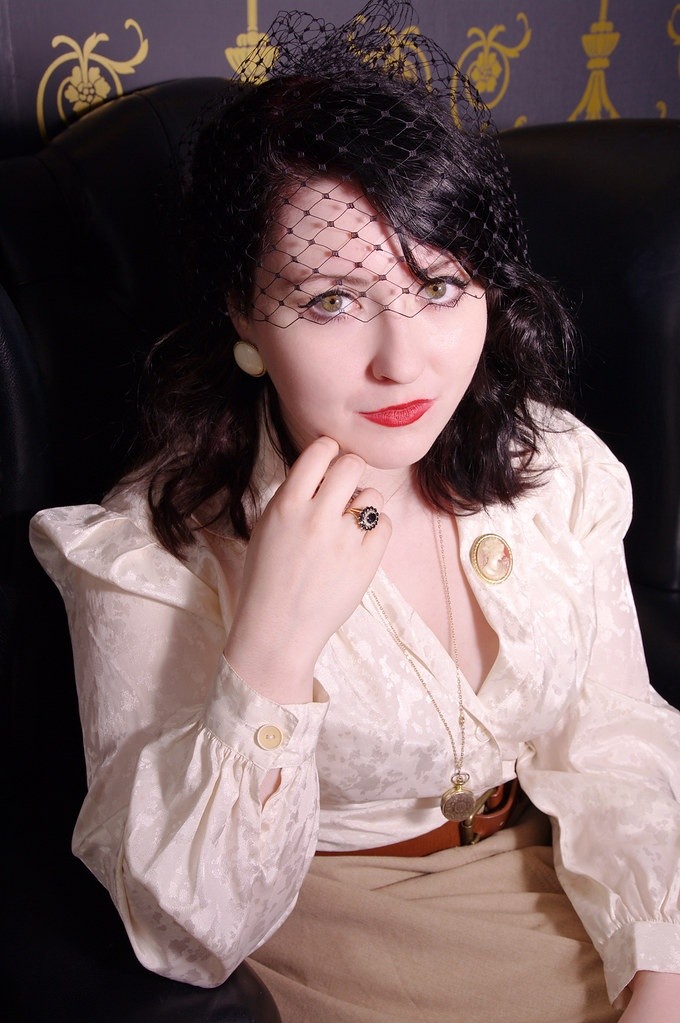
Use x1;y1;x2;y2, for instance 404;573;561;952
344;505;379;531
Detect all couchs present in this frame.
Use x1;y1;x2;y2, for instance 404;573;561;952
0;79;680;1023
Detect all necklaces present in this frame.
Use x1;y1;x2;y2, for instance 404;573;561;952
369;507;476;823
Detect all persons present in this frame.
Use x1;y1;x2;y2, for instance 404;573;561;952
24;0;680;1023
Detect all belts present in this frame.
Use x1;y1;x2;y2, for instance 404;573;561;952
312;777;527;858
